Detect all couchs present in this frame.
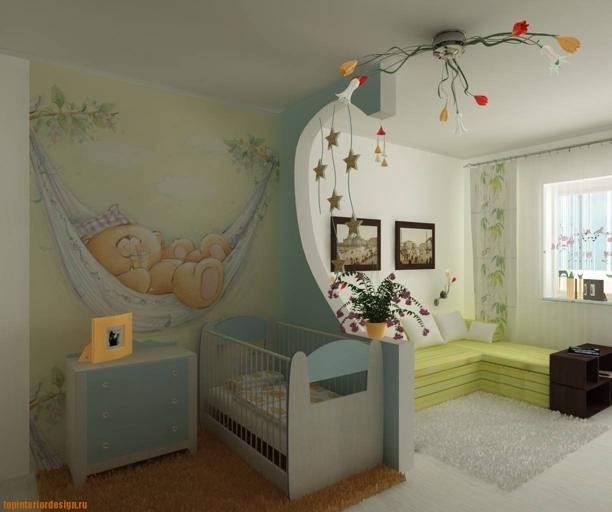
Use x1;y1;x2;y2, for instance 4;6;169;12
406;319;559;412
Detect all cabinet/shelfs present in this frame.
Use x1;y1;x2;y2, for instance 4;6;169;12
549;342;612;419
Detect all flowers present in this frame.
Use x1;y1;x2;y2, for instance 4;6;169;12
328;271;452;340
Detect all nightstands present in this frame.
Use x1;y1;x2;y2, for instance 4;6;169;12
61;346;198;488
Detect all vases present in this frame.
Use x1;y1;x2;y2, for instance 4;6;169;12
366;320;388;338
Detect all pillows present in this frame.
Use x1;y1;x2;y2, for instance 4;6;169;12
384;322;408;341
468;321;499;343
433;312;468;342
401;315;444;350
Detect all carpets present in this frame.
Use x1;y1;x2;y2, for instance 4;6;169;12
37;431;406;512
413;391;608;491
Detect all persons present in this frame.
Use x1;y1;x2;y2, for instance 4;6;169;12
108;331;120;347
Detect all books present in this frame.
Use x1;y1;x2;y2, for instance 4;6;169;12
567;345;601;357
598;369;612;379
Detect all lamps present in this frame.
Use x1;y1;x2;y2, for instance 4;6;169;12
335;20;580;168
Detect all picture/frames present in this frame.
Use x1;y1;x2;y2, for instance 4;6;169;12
330;216;381;272
395;221;435;270
78;313;132;364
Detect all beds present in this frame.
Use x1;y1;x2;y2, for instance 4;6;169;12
199;314;383;501
